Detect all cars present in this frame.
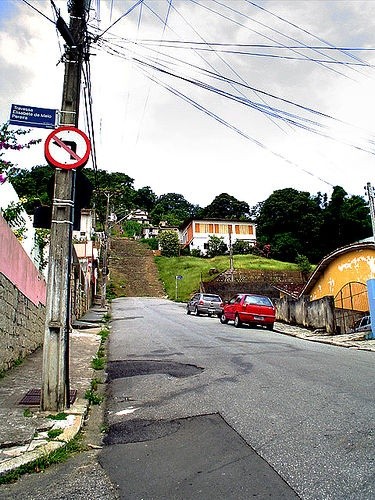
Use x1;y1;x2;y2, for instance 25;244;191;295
221;292;275;330
186;293;226;318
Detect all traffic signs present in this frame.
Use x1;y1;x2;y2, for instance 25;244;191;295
43;125;90;171
